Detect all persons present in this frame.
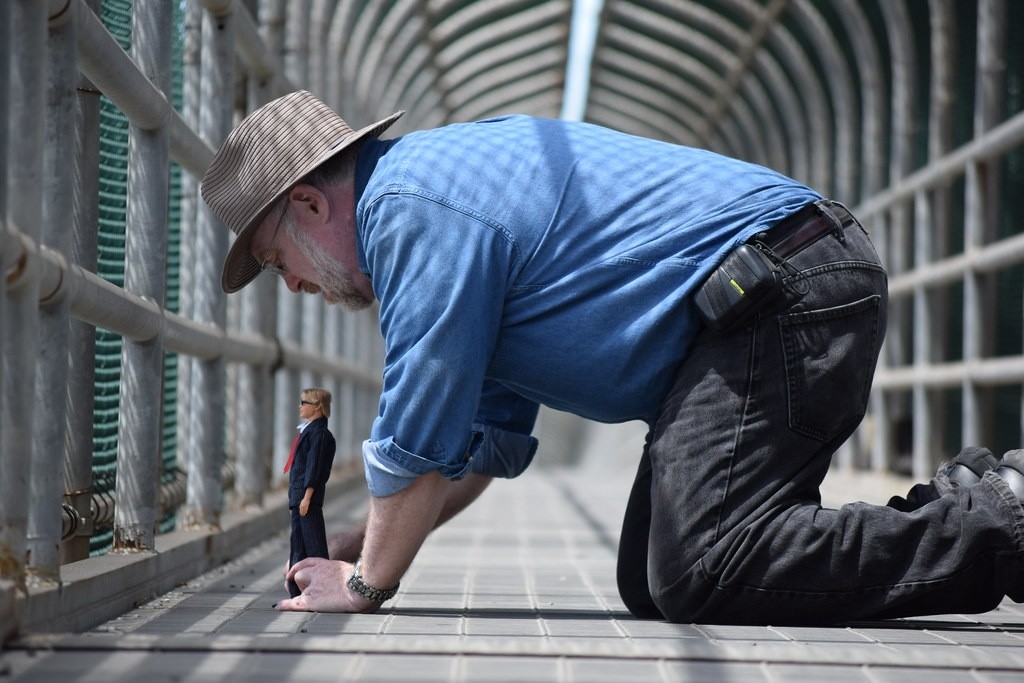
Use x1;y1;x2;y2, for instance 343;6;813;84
201;89;1024;627
284;387;336;612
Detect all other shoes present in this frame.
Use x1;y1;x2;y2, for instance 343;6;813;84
945;445;1024;603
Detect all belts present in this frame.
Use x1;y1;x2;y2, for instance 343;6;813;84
770;202;853;260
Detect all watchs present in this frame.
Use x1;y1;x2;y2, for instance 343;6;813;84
346;556;400;603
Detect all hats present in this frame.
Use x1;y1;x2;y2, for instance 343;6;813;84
201;90;406;294
